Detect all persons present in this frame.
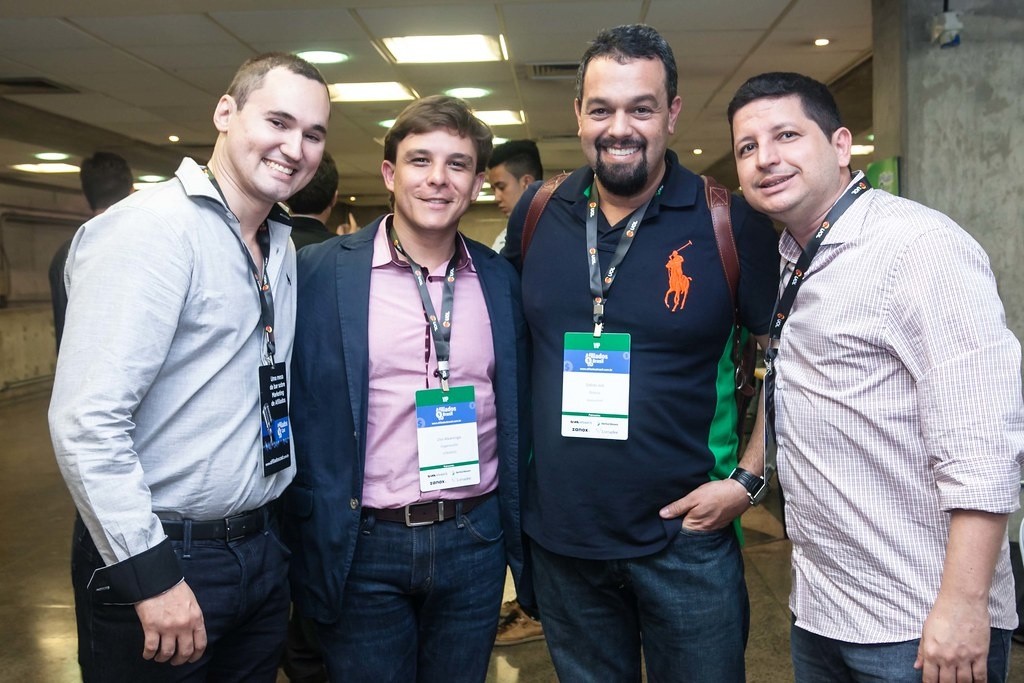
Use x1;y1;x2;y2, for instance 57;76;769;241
410;599;418;601
499;23;779;683
47;51;331;682
49;151;134;356
291;93;532;683
487;139;542;255
730;71;1024;683
285;150;361;251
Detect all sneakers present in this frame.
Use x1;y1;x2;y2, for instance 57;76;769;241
494;604;546;645
500;599;521;618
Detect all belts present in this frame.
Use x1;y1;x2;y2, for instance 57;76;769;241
161;501;273;543
360;488;499;527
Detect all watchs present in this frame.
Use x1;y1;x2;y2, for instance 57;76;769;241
730;468;771;504
